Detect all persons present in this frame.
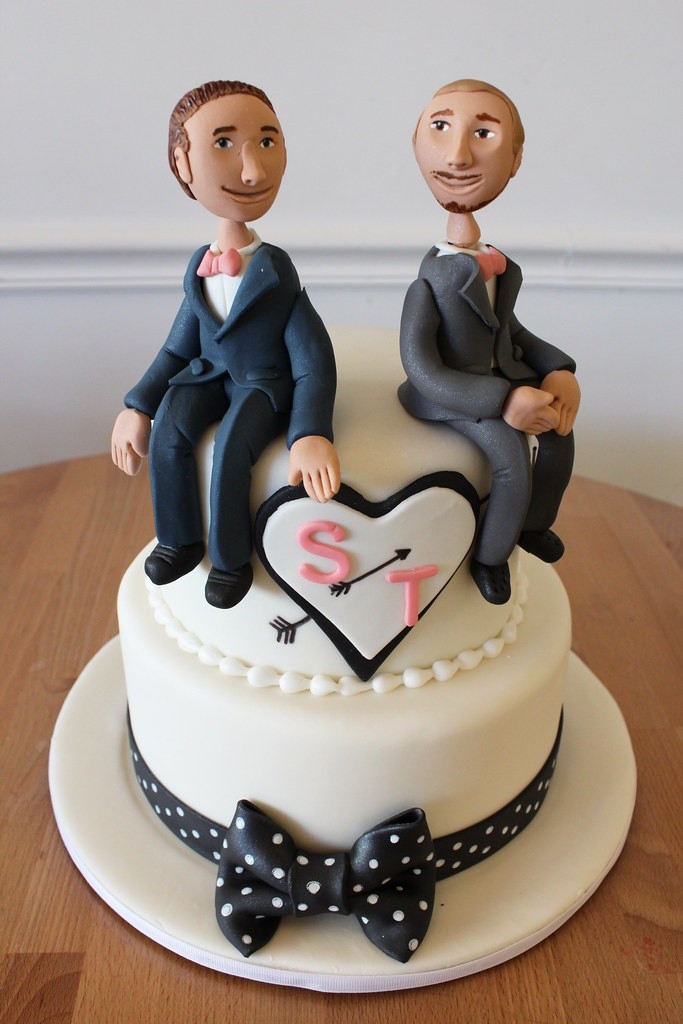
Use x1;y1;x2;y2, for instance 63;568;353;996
397;75;580;606
107;77;344;610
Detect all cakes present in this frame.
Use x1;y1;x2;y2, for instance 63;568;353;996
115;77;582;966
